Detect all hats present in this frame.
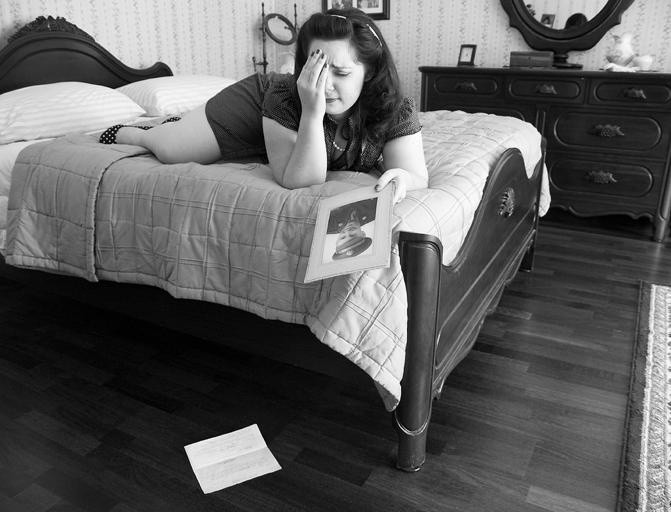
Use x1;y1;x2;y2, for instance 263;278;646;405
332;236;372;260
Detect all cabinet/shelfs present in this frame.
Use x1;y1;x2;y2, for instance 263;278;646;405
419;63;671;251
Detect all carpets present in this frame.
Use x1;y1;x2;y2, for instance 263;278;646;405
611;275;671;509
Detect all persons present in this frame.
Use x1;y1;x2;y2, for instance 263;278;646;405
323;197;378;264
97;5;432;208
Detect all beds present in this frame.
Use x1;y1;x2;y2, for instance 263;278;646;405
2;14;549;475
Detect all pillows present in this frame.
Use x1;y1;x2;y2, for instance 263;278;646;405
3;78;149;146
113;74;242;119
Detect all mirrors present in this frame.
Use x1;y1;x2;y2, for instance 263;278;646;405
498;0;639;68
264;13;297;46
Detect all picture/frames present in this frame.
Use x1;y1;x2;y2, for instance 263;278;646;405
322;0;390;21
303;182;392;286
456;42;476;67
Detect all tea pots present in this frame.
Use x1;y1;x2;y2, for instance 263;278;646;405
605;29;635;67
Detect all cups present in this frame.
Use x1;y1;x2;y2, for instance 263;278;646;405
632;53;655;71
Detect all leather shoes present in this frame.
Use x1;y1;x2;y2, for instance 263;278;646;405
98;115;182;144
